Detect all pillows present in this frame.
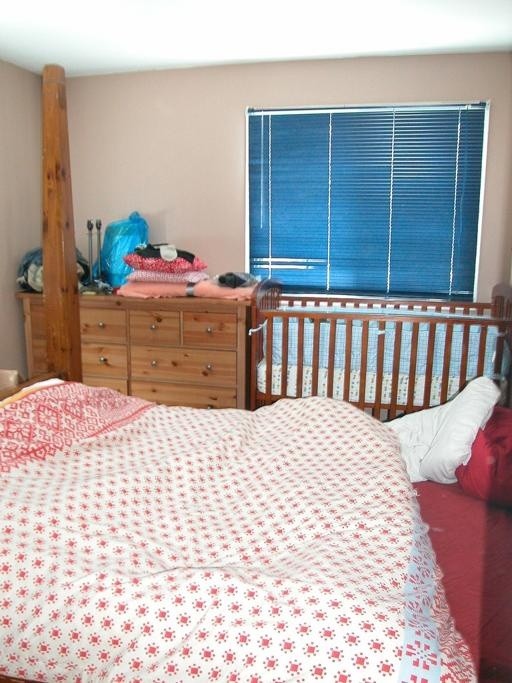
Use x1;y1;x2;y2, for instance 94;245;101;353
381;373;512;506
125;244;209;287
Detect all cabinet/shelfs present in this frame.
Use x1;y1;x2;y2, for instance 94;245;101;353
13;287;247;409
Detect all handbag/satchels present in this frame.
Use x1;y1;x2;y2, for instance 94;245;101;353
16;244;90;292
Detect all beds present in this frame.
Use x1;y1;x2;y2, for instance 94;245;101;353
248;275;512;422
1;364;512;682
